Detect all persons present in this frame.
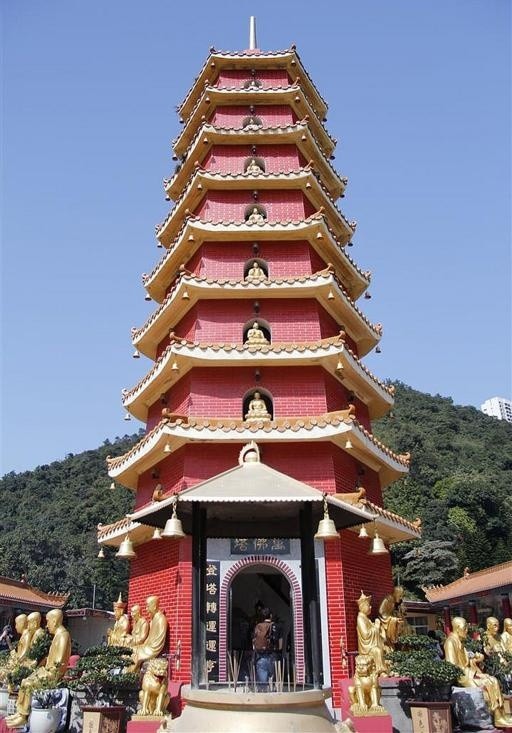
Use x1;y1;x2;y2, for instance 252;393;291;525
352;589;402;680
4;608;73;730
247;392;269;414
441;615;511;728
249;607;279;689
6;613;38;670
0;623;16;653
481;615;507;667
246;262;267;278
247;159;260;171
379;584;412;641
249;207;264;221
105;599;131;648
248;80;258;89
245;118;258;127
122;605;150;649
8;610;46;693
242;599;268;642
499;616;511;656
131;595;169;672
247;322;265;339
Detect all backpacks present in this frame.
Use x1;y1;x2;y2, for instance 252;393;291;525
254;622;274;653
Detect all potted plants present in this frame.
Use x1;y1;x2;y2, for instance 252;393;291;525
64;672;140;733
485;651;512;715
389;656;465;733
20;660;79;733
0;665;35;717
